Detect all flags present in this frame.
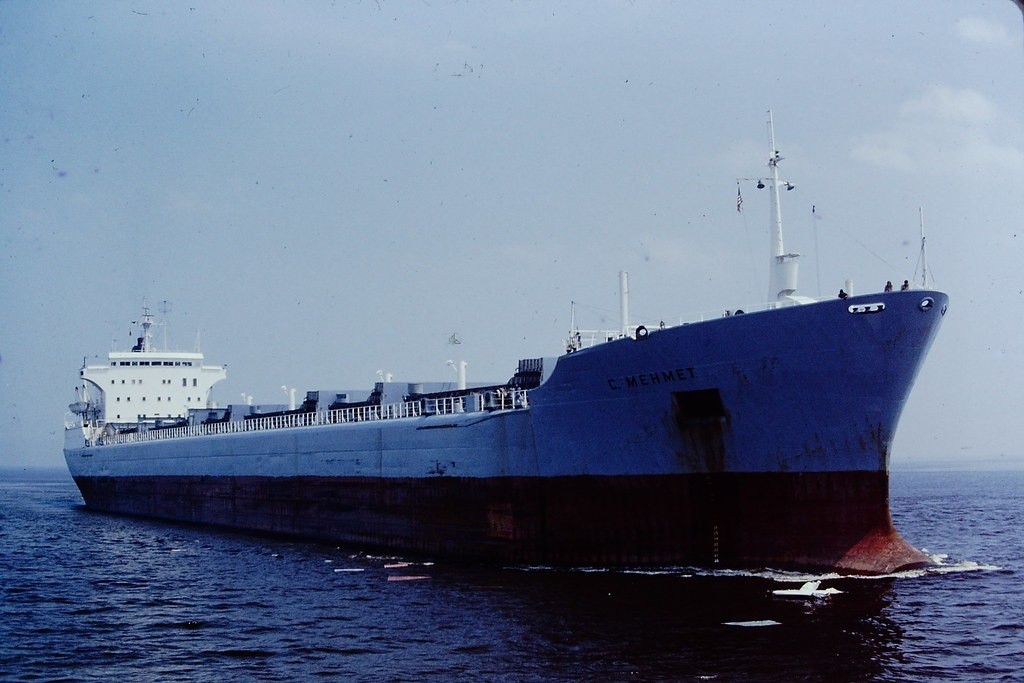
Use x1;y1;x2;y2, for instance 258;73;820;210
737;185;743;212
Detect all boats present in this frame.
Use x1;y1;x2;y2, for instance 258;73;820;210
62;108;950;575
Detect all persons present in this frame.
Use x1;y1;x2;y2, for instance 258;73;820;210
884;281;893;291
838;289;847;298
901;280;909;290
